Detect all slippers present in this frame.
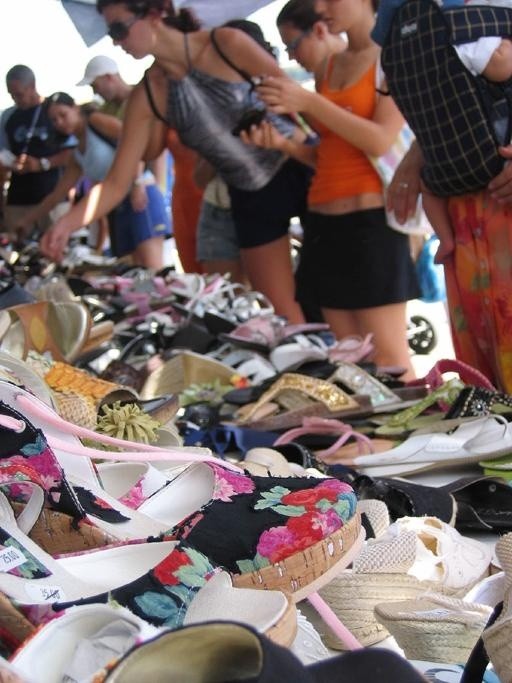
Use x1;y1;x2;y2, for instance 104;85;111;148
0;231;512;682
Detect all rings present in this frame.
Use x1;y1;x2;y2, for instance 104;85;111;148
400;183;408;187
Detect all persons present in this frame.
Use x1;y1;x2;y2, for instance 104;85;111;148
40;2;326;325
0;57;215;274
371;1;512;394
253;0;421;381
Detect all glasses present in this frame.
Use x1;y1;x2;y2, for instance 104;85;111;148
106;13;142;41
285;32;306;52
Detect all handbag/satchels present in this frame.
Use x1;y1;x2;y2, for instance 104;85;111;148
366;52;428;235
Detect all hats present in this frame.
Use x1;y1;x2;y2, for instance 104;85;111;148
75;56;118;86
